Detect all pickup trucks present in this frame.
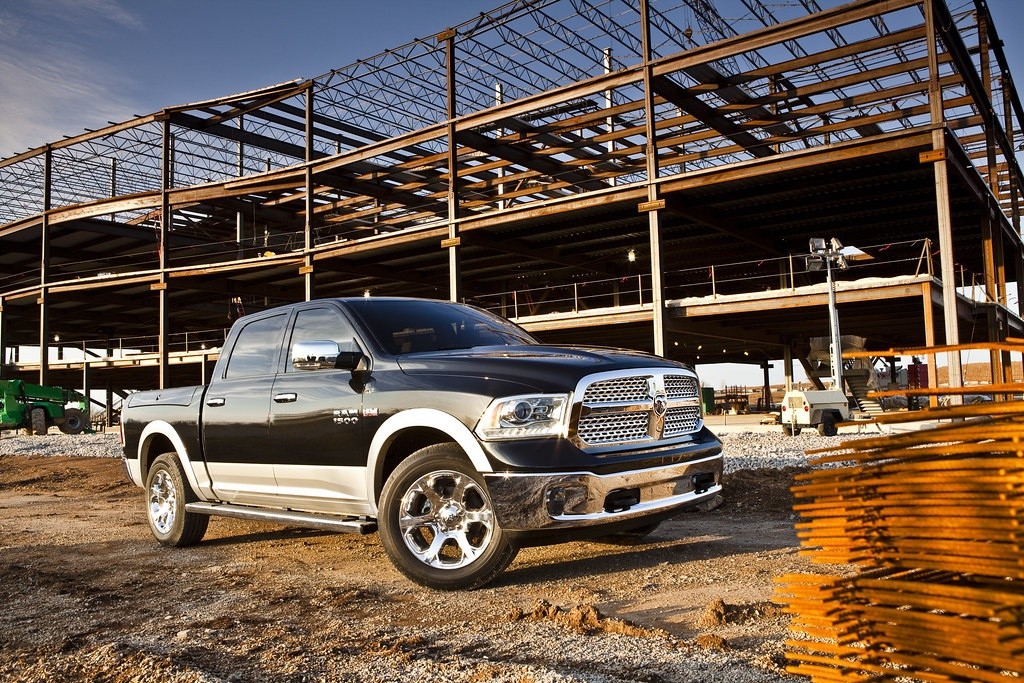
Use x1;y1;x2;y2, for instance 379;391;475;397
120;297;725;592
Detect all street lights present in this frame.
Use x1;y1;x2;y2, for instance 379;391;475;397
805;237;850;390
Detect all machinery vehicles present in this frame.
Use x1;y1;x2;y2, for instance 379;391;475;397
0;379;96;435
